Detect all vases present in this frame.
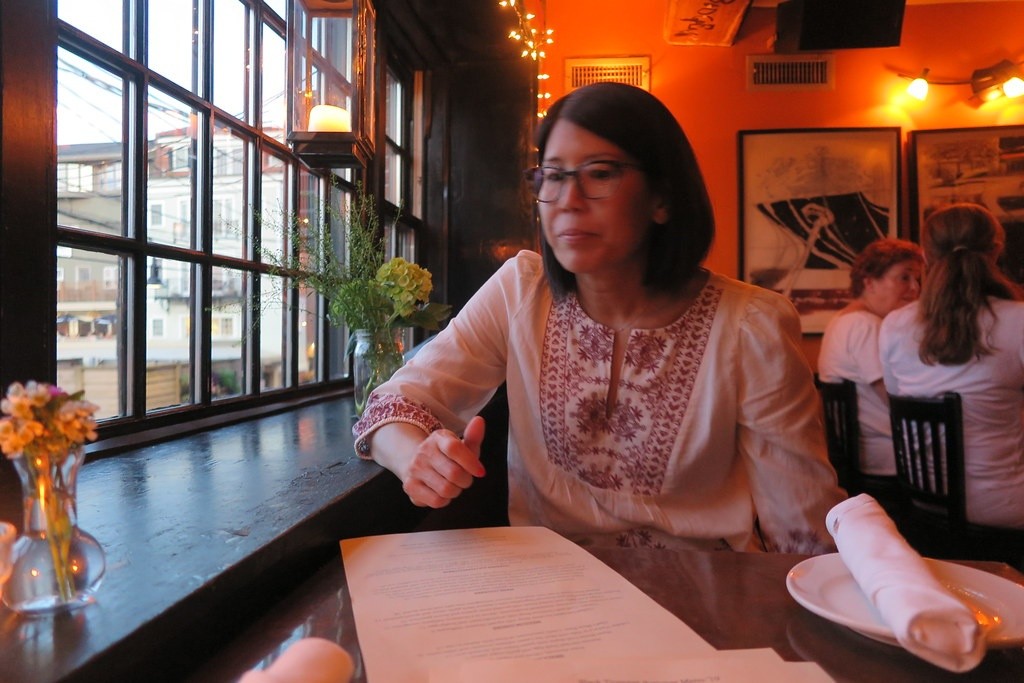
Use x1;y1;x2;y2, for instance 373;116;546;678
0;448;105;621
353;329;405;420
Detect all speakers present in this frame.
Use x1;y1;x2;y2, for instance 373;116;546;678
774;0;906;53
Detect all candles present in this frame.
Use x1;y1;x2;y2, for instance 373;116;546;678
308;106;350;132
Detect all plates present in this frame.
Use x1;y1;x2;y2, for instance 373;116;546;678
787;551;1024;653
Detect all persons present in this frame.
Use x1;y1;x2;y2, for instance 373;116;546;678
876;203;1024;532
818;238;925;498
352;82;849;553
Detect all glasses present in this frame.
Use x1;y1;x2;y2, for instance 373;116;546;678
521;160;641;203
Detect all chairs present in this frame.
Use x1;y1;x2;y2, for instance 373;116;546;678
812;371;899;501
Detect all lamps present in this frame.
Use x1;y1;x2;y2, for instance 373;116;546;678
909;67;931;100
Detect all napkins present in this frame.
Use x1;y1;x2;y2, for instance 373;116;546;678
827;491;985;675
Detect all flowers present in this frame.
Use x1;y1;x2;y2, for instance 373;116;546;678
202;169;453;415
0;383;101;603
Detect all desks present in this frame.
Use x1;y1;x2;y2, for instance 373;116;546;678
886;389;1024;564
150;537;1024;683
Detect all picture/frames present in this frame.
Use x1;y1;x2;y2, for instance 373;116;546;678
910;125;1024;298
738;127;901;335
